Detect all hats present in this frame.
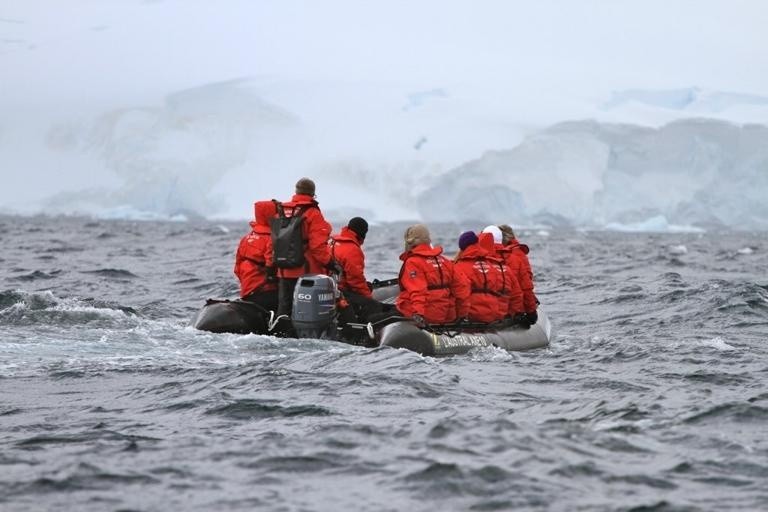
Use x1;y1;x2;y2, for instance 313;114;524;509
295;177;315;195
348;217;368;240
405;225;431;246
459;225;514;248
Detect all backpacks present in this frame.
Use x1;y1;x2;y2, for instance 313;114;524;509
271;199;319;268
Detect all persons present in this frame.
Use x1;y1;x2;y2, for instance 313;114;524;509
262;177;341;318
361;224;472;334
326;217;391;327
480;225;509;268
234;198;282;314
497;223;541;327
450;230;531;331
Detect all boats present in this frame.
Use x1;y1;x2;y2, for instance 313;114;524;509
188;278;552;357
